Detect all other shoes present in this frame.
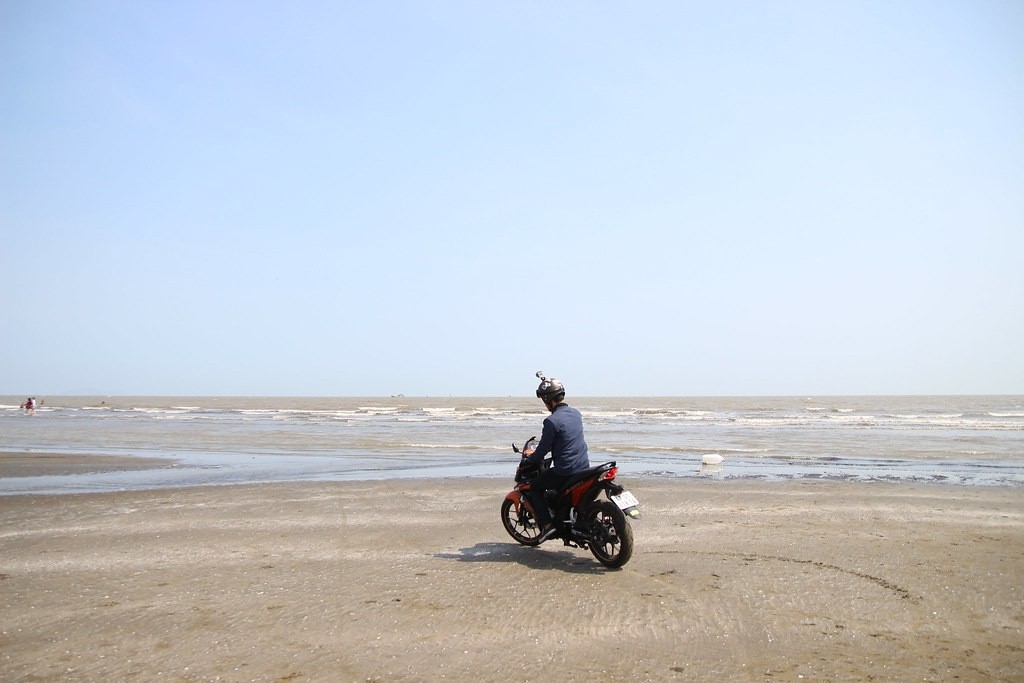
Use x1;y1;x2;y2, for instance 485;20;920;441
539;525;557;544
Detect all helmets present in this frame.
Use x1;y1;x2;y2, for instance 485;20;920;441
536;371;565;404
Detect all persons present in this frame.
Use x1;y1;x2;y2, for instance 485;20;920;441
516;376;590;543
24;396;36;416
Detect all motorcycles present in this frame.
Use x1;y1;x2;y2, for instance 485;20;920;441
499;435;640;569
